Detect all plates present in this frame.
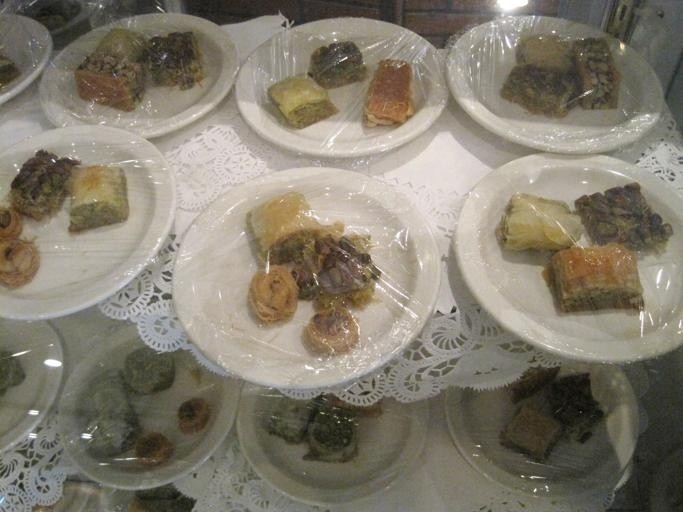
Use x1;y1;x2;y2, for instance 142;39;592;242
445;12;667;156
1;123;176;322
169;165;447;392
453;148;681;364
233;16;451;161
442;351;642;498
1;319;68;453
34;10;239;141
0;12;55;109
232;368;429;509
52;323;242;492
